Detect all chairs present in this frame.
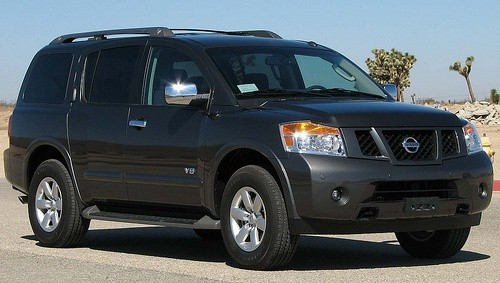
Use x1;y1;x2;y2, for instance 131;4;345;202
243;73;269;92
184;76;210;94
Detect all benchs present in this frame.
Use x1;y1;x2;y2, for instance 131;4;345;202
92;69;187;103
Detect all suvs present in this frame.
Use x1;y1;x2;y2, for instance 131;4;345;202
2;26;495;271
